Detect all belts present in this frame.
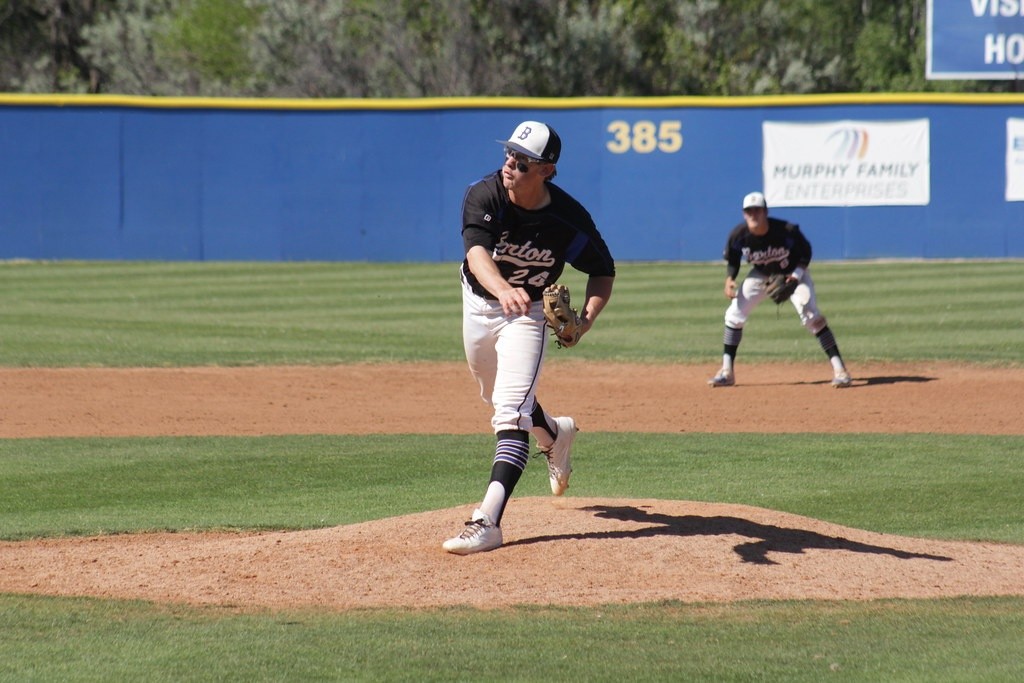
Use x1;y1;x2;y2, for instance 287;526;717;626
460;274;542;302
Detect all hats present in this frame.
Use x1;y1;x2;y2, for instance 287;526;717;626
742;192;767;209
495;120;562;164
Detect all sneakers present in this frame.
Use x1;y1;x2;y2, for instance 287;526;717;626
441;508;503;555
531;416;580;495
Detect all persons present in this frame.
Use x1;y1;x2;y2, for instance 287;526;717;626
443;121;615;557
707;192;851;389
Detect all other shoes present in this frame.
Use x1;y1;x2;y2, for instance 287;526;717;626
831;372;851;387
707;376;735;385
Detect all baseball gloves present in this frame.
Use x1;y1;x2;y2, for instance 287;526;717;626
543;284;581;348
765;275;799;304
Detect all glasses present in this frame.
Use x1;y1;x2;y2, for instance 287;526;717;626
503;150;545;164
